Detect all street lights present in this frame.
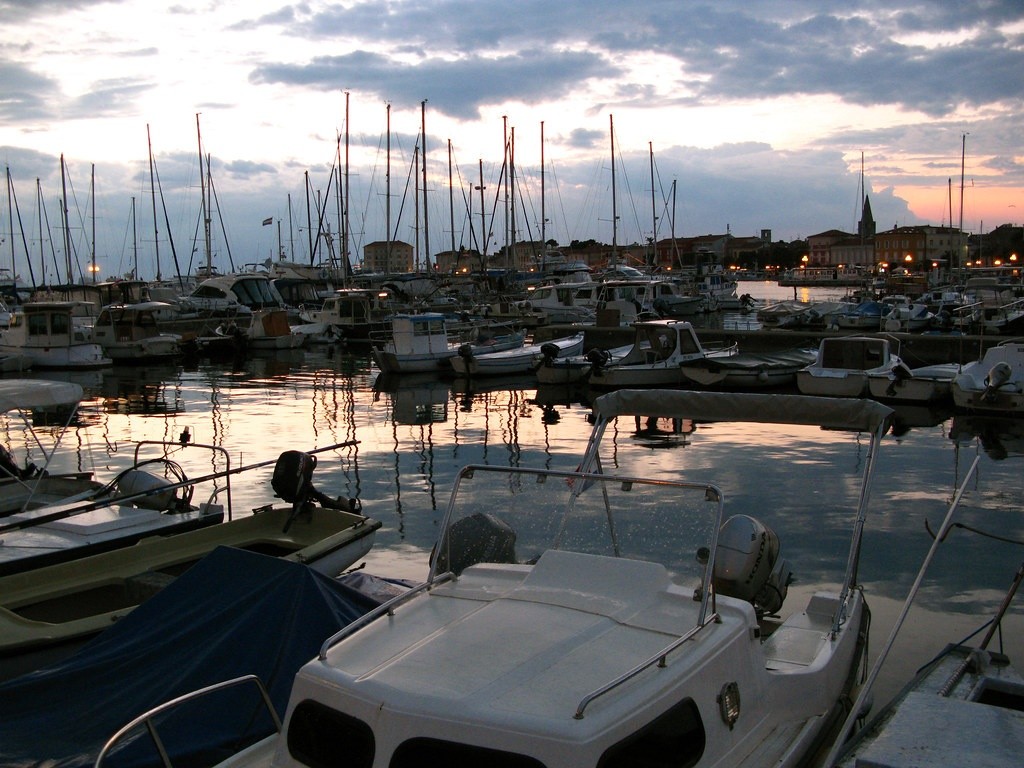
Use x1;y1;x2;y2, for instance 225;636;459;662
802;256;808;279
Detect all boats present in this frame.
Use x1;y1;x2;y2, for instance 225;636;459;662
0;441;232;575
886;304;934;328
0;379;116;518
935;303;981;325
951;343;1024;412
368;310;525;373
581;320;738;387
869;361;964;400
532;335;667;384
449;331;585;376
838;301;882;326
0;450;382;651
95;389;896;768
801;302;858;329
797;332;903;396
679;347;818;387
756;301;813;327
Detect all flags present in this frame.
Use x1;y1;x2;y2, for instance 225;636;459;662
262;217;272;226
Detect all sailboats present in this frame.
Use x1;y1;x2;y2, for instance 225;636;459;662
0;93;391;370
916;134;969;304
392;100;758;322
